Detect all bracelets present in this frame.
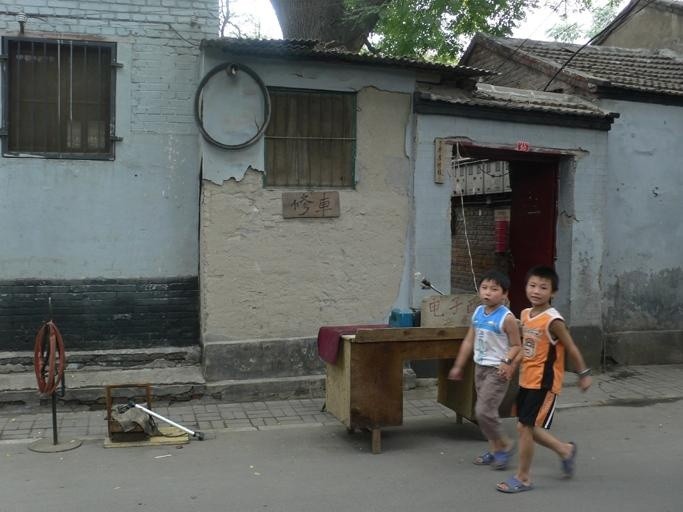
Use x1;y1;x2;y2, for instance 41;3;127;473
576;368;591;377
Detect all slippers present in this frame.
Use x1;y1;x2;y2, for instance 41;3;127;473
473;443;518;471
562;441;576;478
496;477;535;493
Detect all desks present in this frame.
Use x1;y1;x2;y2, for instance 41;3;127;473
323;326;524;454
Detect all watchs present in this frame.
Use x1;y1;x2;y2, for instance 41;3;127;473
501;357;511;366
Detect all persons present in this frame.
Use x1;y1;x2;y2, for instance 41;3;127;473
496;265;592;493
447;270;520;470
